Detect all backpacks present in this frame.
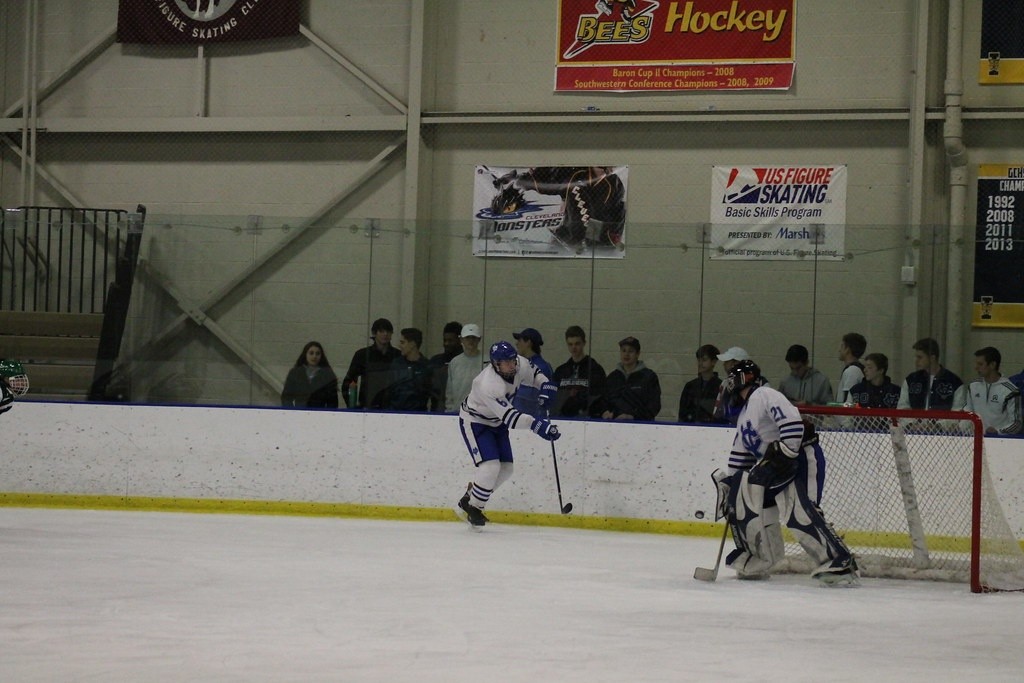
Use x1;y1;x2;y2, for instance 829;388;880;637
841;362;875;406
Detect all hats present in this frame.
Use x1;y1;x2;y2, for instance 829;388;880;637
717;347;750;361
513;328;543;344
458;324;482;337
619;337;640;352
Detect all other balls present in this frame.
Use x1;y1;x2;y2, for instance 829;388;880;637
695;510;704;519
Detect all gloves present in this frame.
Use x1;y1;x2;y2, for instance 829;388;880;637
537;382;558;407
531;417;561;440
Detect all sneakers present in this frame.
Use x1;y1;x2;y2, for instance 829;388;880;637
454;482;473;522
466;505;490;532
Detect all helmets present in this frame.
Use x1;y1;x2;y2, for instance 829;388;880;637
490;341;517;360
728;361;761;395
0;360;30;398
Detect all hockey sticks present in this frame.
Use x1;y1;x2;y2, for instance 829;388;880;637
541;398;575;515
693;465;746;582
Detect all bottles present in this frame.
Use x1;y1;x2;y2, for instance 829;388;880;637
825;401;860;407
348;381;357;408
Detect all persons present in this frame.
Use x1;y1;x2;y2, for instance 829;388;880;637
778;345;836;431
678;339;749;427
556;326;607;422
511;327;558;419
376;327;444;414
716;359;858;583
0;356;29;414
341;318;401;412
280;342;340;411
429;322;464;417
846;353;902;433
896;338;967;435
457;341;561;528
446;324;486;414
604;336;661;423
952;346;1024;438
1009;366;1024;439
833;333;864;431
